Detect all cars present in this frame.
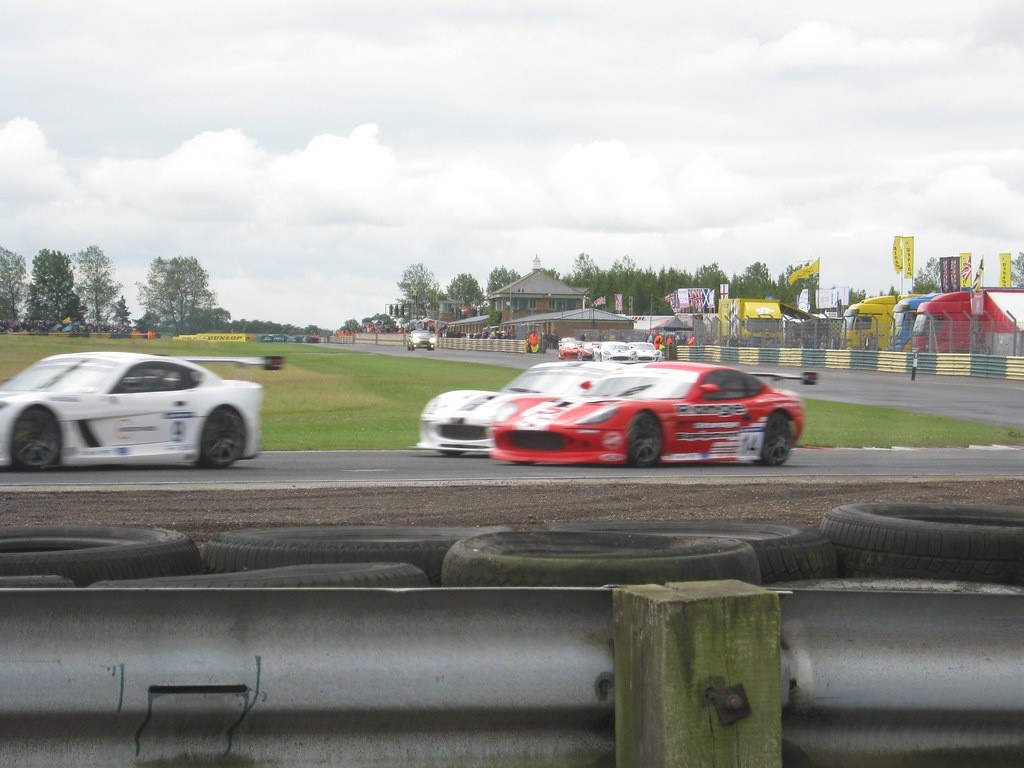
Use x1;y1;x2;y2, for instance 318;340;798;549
415;360;805;468
1;351;264;471
405;329;438;351
558;335;664;364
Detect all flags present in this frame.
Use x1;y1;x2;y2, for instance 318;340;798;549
788;260;819;285
594;297;605;306
688;289;714;308
720;284;728;299
973;259;983;292
999;253;1010;288
666;291;676;301
893;237;914;279
940;253;972;293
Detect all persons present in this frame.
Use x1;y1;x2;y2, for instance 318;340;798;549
528;331;558;353
0;319;149;337
581;334;585;341
675;333;695;345
863;333;874;350
467;329;511;339
599;332;618;341
701;332;781;348
800;333;840;349
648;333;673;349
336;321;435;337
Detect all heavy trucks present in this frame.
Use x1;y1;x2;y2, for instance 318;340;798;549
840;288;1024;357
717;298;785;350
